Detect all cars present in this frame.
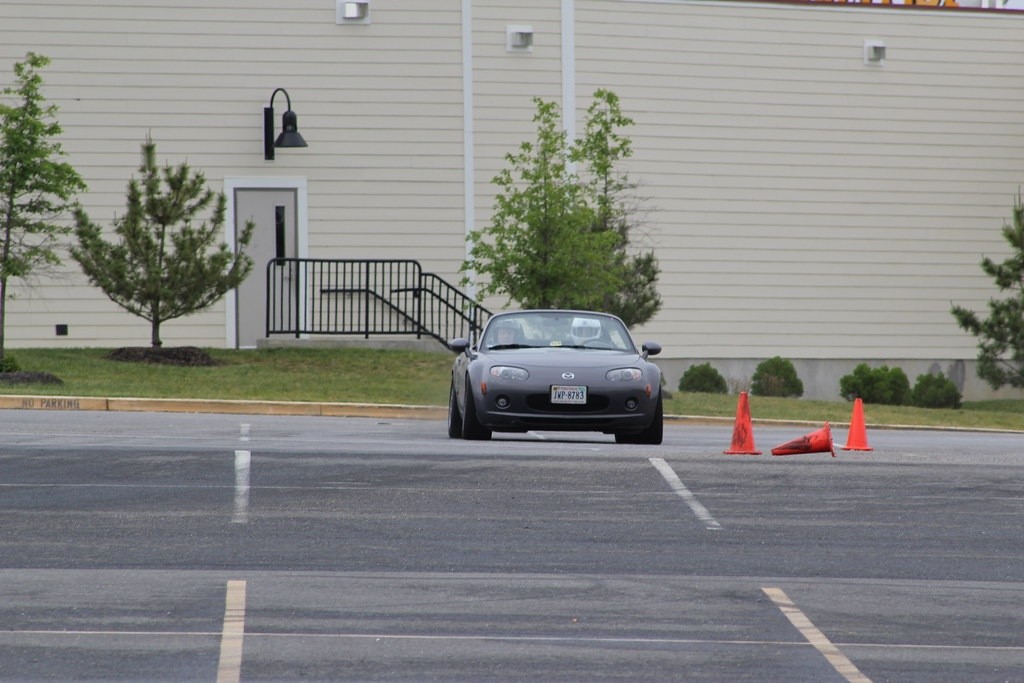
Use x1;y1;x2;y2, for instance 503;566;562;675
446;309;667;445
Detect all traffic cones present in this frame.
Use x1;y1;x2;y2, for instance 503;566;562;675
840;397;875;452
723;394;764;456
771;421;836;457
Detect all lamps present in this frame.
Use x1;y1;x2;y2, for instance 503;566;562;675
263;87;309;160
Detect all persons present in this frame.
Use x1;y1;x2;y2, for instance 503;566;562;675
562;317;615;350
492;320;523;346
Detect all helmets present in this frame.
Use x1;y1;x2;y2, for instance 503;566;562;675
571;317;601;345
491;318;520;344
